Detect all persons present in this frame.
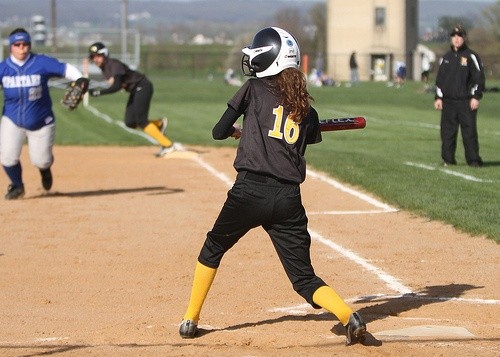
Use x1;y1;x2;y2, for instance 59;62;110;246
434;27;485;168
86;42;174;156
0;28;89;200
225;48;432;88
178;26;366;347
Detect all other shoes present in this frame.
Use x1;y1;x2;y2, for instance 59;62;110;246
40;172;52;190
155;142;176;158
159;117;167;132
6;182;24;198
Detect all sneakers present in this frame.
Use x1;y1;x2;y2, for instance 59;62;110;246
178;319;197;339
342;312;366;347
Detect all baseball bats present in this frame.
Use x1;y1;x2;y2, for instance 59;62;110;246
239;117;366;136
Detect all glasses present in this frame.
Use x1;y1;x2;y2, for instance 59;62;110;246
12;42;30;46
450;33;463;36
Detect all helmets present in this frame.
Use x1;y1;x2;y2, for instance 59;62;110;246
241;27;300;77
89;42;109;60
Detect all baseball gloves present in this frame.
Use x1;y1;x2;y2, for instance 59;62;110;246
62;77;90;112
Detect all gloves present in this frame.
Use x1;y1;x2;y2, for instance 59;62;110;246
88;87;101;97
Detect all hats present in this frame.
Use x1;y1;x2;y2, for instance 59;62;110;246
451;24;465;34
